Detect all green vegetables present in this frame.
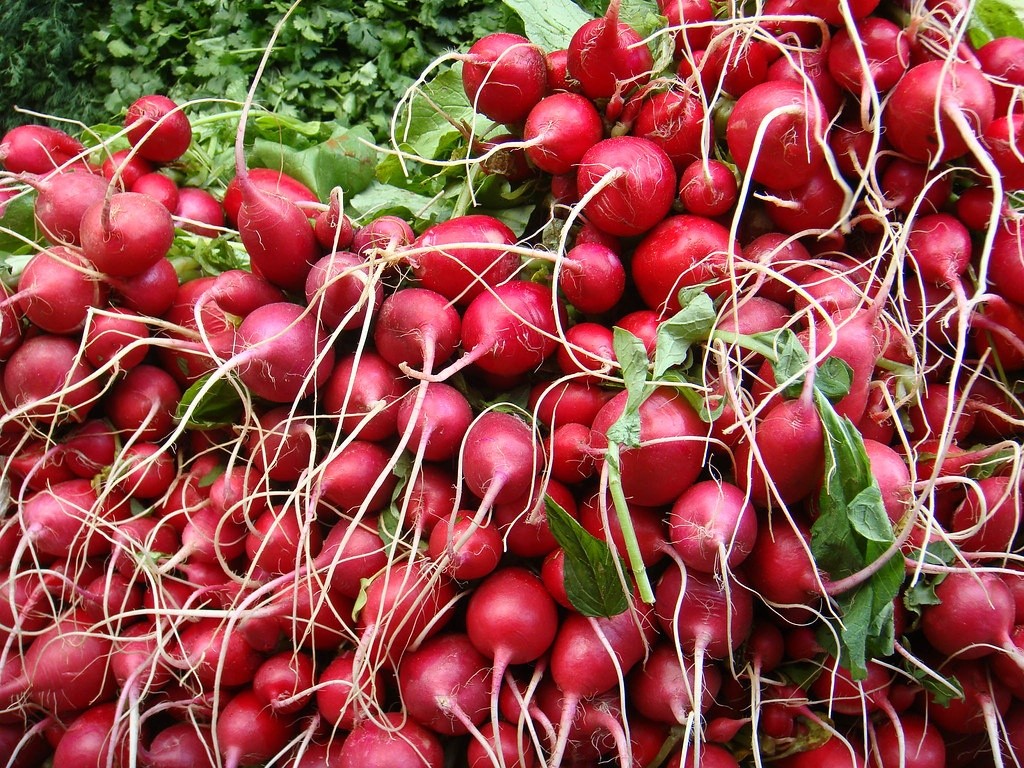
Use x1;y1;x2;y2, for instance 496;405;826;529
0;0;609;148
